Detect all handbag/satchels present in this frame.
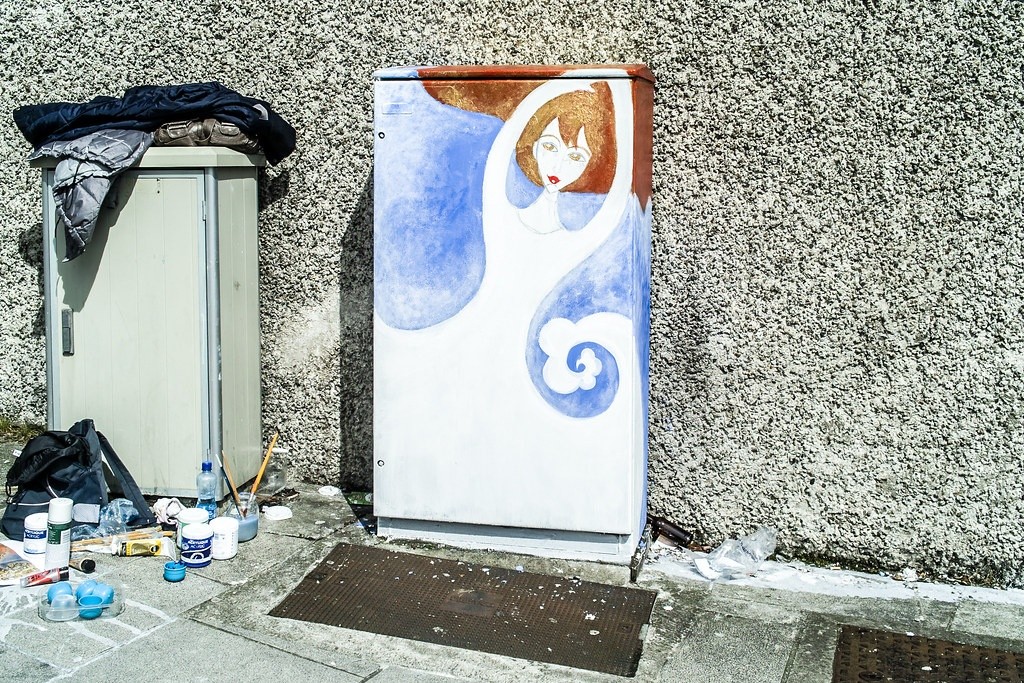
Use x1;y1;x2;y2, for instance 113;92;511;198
154;119;264;155
0;419;157;542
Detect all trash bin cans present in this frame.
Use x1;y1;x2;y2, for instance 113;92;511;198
21;144;262;508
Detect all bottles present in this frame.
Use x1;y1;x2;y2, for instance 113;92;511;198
647;514;693;546
195;461;217;520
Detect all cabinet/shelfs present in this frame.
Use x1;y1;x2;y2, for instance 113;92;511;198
32;146;268;500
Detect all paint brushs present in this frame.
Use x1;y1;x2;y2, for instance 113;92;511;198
214;431;278;520
